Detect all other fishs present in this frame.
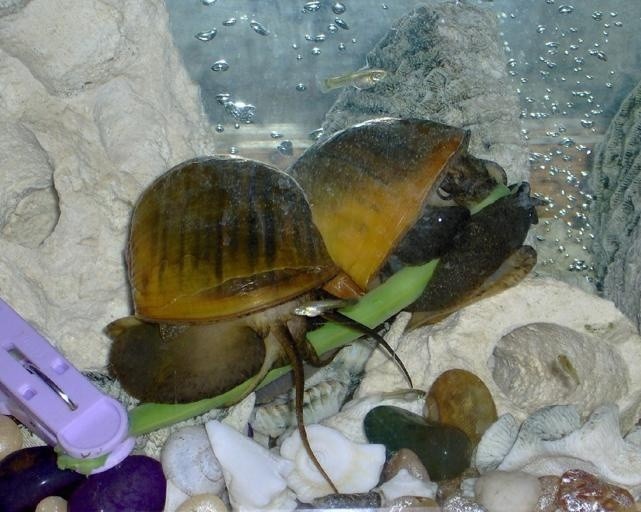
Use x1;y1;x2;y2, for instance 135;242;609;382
318;69;386;94
554;352;580;391
292;298;349;318
368;386;426;407
